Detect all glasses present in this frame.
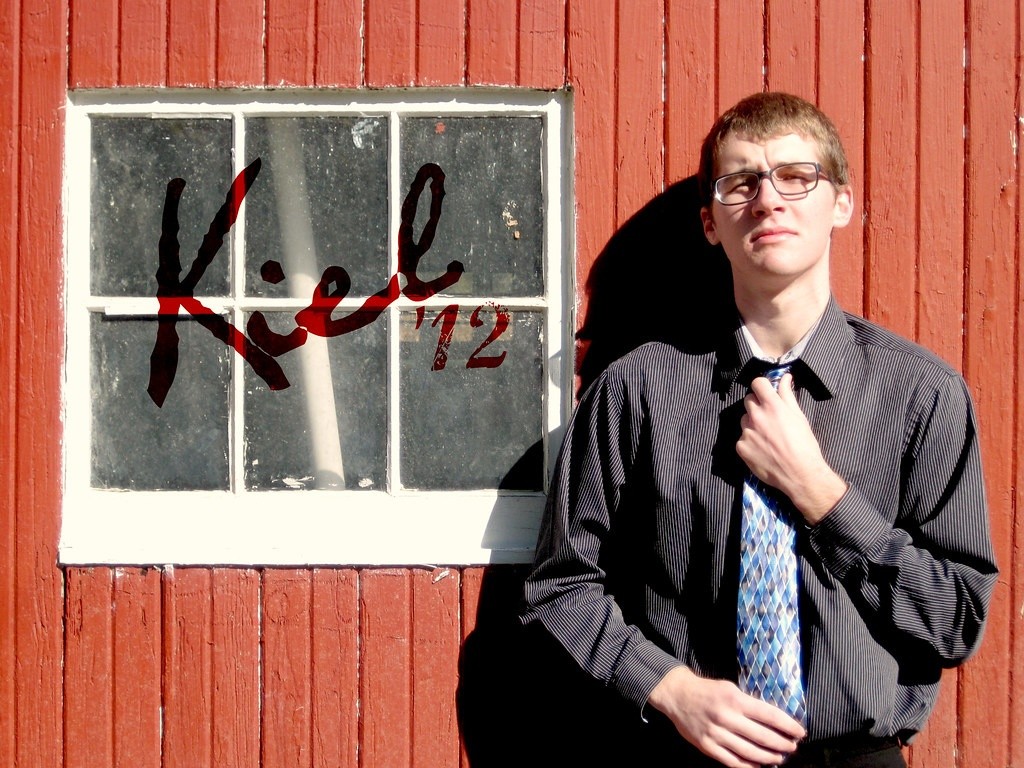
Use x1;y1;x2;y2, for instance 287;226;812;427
711;162;841;206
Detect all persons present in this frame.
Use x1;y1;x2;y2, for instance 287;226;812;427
523;91;999;768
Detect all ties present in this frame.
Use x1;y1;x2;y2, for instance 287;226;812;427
736;366;805;721
